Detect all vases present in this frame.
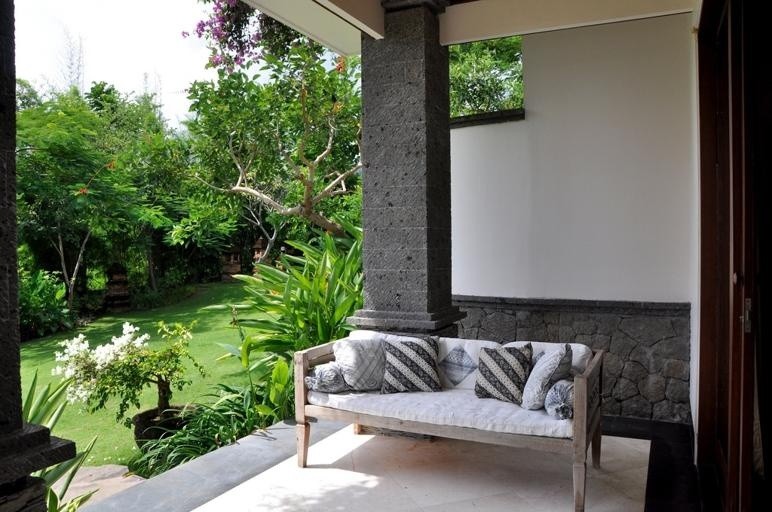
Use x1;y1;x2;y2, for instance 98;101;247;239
131;406;202;454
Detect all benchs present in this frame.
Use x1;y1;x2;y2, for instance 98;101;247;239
294;331;607;512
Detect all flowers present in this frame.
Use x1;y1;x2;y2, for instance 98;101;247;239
51;320;210;427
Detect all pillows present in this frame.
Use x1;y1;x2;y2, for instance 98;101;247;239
305;332;576;418
